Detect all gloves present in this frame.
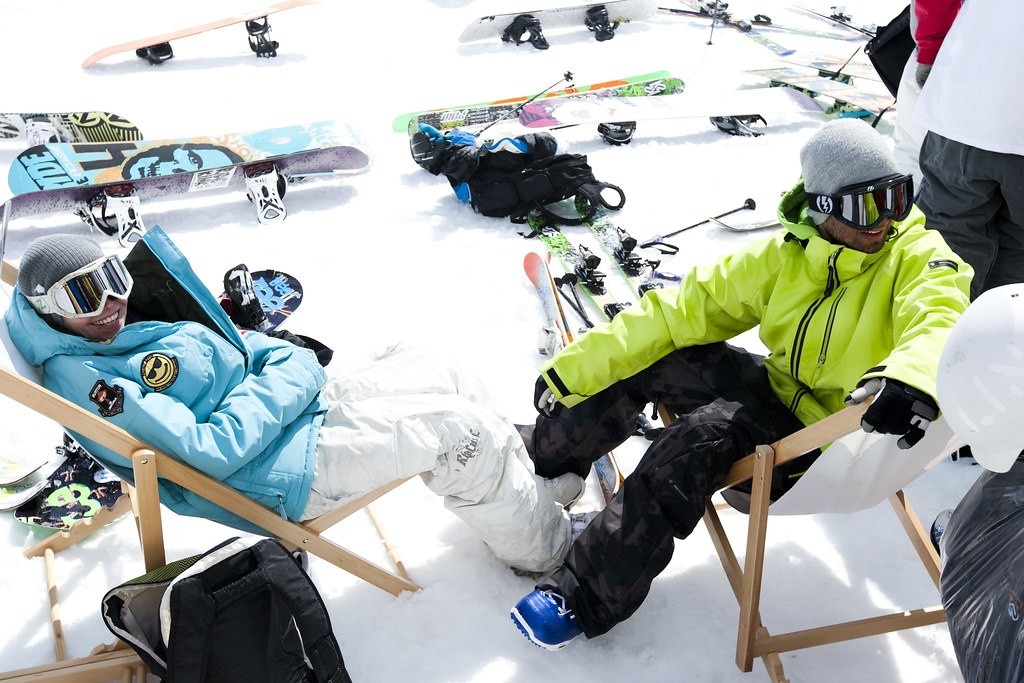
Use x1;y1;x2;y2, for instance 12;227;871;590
534;374;563;420
857;376;940;451
916;63;932;88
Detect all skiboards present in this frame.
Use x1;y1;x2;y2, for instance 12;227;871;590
390;68;686;138
526;192;662;321
670;0;876;56
525;250;626;507
708;216;779;232
1;453;51;514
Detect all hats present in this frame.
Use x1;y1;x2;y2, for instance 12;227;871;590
17;234;104;301
800;116;908;224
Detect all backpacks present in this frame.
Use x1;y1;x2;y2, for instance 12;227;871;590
102;535;352;683
443;128;624;223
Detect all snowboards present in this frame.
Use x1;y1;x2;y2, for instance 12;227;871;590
80;1;316;70
743;56;896;117
7;119;371;199
517;85;835;130
0;145;369;228
458;0;660;45
0;109;144;142
15;267;304;532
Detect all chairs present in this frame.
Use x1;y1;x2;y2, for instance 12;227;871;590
0;368;418;683
654;390;950;683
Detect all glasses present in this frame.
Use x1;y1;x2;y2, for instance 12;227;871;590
806;172;914;232
23;253;134;319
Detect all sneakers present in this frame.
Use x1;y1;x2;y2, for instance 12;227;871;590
572;511;602;544
509;587;585;653
545;473;585;508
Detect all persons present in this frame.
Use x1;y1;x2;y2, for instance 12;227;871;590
4;236;603;579
892;0;1024;303
511;118;975;653
